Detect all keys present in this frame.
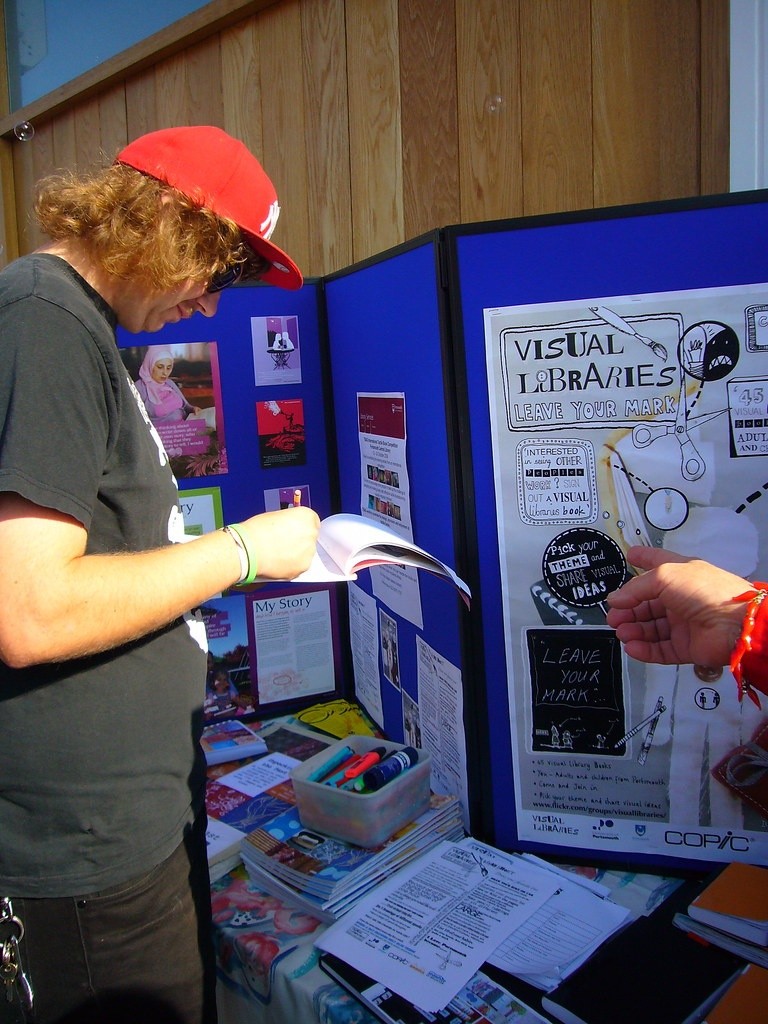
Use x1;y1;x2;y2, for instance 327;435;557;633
0;894;38;1024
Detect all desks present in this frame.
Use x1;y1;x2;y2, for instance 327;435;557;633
204;864;685;1024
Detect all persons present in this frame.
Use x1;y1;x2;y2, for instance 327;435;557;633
134;344;203;424
0;121;322;1024
605;544;768;712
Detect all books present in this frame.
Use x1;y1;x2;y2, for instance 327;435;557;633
201;720;466;926
672;860;768;969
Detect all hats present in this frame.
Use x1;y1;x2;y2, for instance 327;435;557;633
115;124;304;291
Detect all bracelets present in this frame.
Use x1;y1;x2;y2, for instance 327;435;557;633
222;522;259;589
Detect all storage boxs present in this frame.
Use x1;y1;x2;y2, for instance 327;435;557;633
290;734;432;847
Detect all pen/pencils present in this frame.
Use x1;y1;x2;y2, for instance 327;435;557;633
293;490;301;507
306;746;419;790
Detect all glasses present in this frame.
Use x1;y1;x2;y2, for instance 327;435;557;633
206;261;242;295
